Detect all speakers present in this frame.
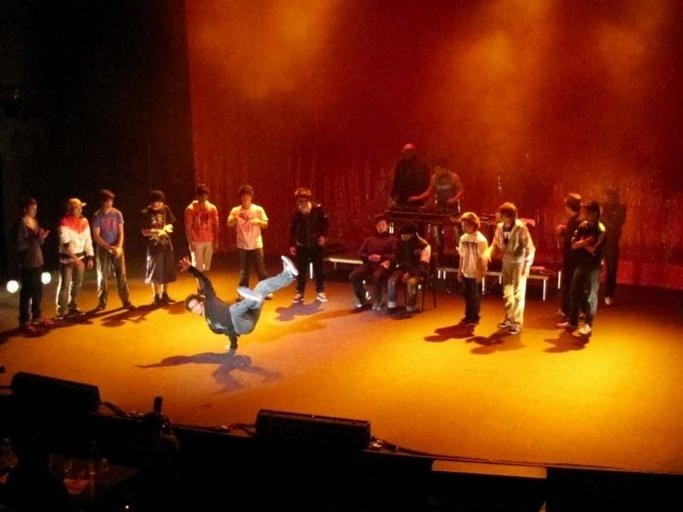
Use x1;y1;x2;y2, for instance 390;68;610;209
10;372;101;414
256;409;370;456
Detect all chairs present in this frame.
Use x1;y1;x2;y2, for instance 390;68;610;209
386;235;438;312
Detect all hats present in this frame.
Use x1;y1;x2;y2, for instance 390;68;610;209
68;197;86;210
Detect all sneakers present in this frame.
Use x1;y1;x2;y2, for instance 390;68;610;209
462;316;481;325
153;298;174;305
281;255;300;276
499;320;522;334
291;292;305;303
20;302;85;333
316;293;329;303
557;308;593;335
361;300;418;315
96;303;105;310
124;302;134;309
238;286;263;301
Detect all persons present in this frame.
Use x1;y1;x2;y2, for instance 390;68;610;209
487;204;536;335
392;145;464;210
226;185;273;301
56;199;94;320
351;216;432;317
140;191;176;304
180;255;298;351
93;189;135;311
184;186;220;272
288;189;331;302
17;199;54;334
556;190;626;337
458;212;489;326
